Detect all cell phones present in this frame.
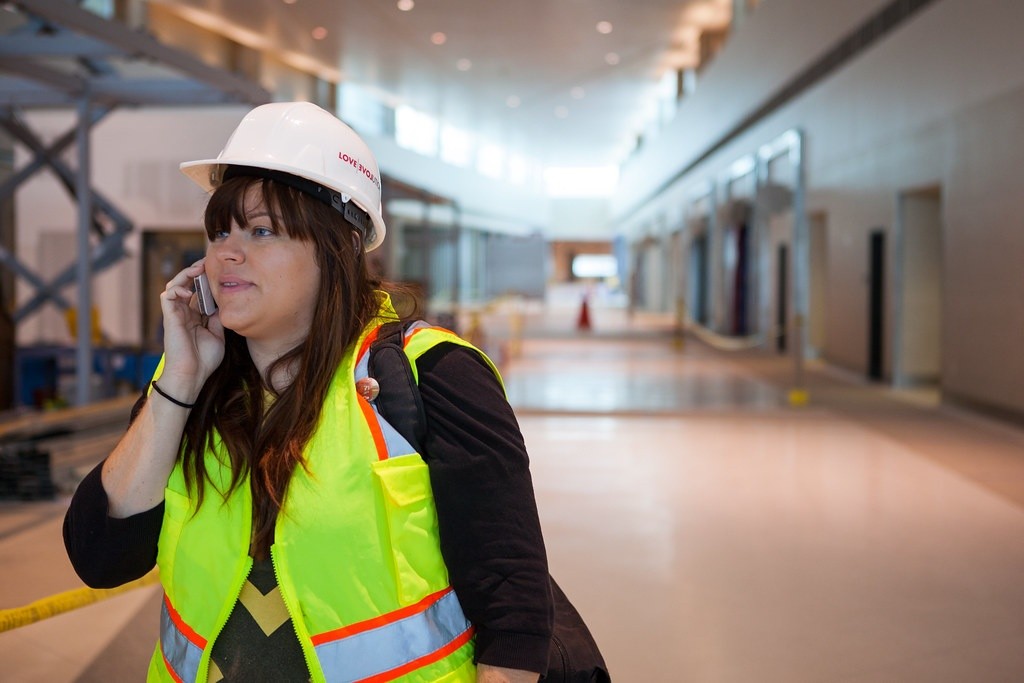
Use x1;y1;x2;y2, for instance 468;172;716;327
193;271;219;316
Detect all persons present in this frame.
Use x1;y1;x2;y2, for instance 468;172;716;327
61;102;554;683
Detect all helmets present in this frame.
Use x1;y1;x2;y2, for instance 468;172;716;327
181;104;388;255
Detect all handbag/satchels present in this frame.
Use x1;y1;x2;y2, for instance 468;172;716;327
537;578;612;683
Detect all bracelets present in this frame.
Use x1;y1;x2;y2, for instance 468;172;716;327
152;381;194;408
